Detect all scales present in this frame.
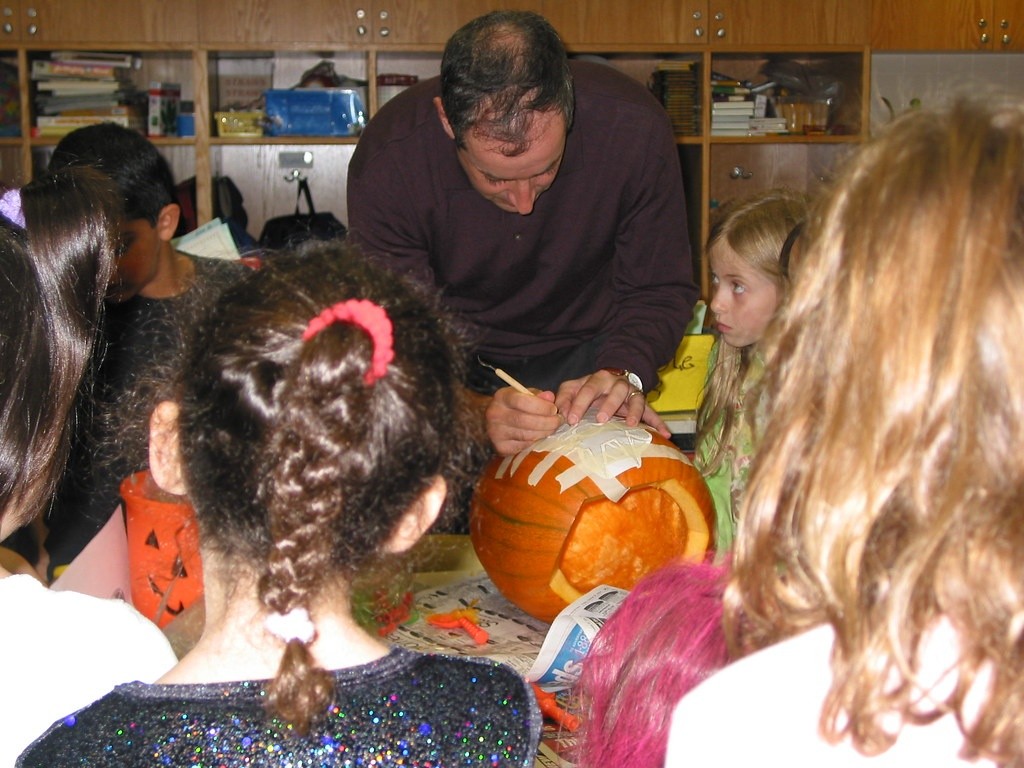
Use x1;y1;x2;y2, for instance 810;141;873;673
645;335;715;449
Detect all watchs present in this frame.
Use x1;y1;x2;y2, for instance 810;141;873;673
624;369;644;392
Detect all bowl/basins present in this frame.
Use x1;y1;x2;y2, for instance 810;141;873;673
774;103;828;135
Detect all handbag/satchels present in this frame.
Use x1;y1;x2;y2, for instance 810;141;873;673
259;178;346;251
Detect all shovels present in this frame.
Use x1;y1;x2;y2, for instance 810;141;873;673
426;614;490;645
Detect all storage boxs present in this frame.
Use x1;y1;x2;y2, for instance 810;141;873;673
264;86;362;139
647;335;715;412
148;83;180;138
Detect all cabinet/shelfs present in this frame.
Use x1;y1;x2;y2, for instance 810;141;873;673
1;0;1024;331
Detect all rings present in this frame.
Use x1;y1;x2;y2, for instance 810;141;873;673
628;390;643;400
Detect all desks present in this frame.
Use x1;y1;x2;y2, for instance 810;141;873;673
358;570;585;768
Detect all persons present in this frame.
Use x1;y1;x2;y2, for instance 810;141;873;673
582;81;1024;768
20;122;255;588
14;245;541;768
346;9;698;534
0;168;180;768
